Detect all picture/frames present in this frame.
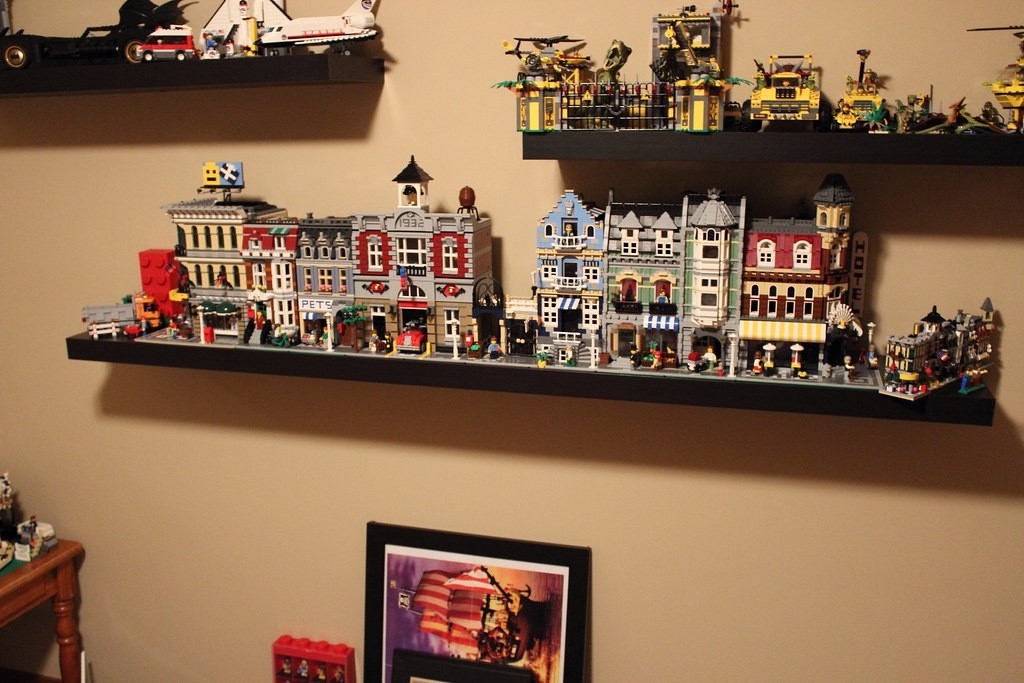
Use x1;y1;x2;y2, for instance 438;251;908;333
364;521;595;683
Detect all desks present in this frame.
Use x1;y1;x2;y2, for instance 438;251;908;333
0;532;84;683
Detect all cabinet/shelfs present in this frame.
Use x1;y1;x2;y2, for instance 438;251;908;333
2;54;1023;423
